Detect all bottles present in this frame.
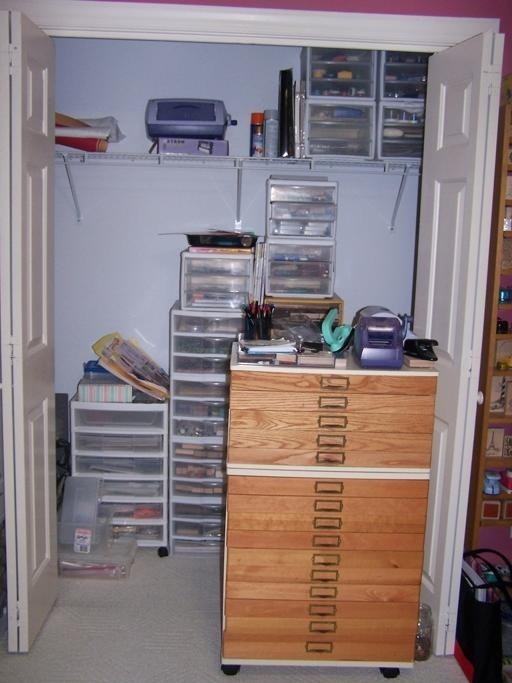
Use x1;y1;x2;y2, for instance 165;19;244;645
250;109;280;160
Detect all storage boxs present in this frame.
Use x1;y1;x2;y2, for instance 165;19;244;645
298;47;436;162
57;536;138;579
60;476;100;545
165;250;256;557
263;177;338;300
57;505;114;553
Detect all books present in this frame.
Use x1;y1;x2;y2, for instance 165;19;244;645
238;337;298;363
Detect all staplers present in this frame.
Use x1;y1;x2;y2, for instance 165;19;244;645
404;339;439;362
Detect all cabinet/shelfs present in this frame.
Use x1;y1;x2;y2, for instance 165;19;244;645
69;390;169;559
465;104;512;568
219;342;440;679
263;294;345;329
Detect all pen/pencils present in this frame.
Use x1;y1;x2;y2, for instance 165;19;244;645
241;301;274;318
